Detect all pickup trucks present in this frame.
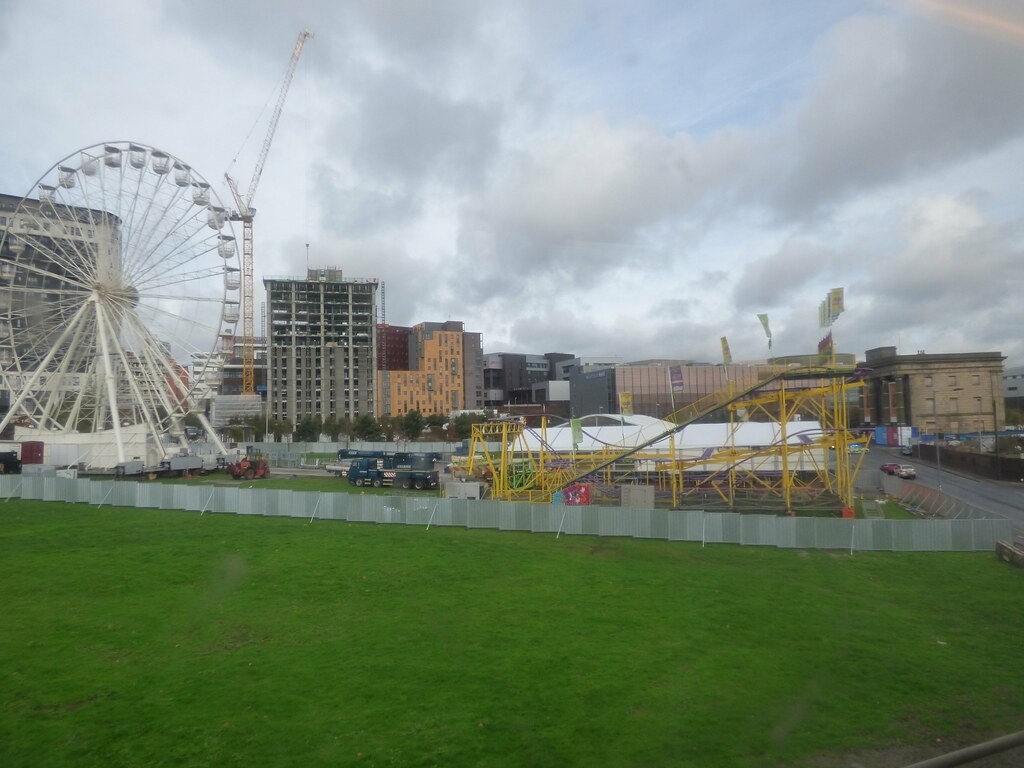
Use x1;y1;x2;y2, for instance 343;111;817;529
899;465;916;479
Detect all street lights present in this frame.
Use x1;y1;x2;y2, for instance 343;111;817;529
654;401;661;419
514;396;518;414
954;387;983;454
599;406;603;414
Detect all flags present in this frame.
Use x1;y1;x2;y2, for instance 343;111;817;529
668;365;684;393
720;335;732;366
818;297;839;328
831;287;844;316
571;418;583;444
758;313;771;337
621;392;635;417
827;293;839;317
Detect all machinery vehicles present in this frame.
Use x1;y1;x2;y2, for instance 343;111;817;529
224;446;270;481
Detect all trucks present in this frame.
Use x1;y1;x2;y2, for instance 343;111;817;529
337;447;441;490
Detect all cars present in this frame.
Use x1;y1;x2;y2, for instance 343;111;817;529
880;463;897;474
944;435;958;441
848;444;869;455
